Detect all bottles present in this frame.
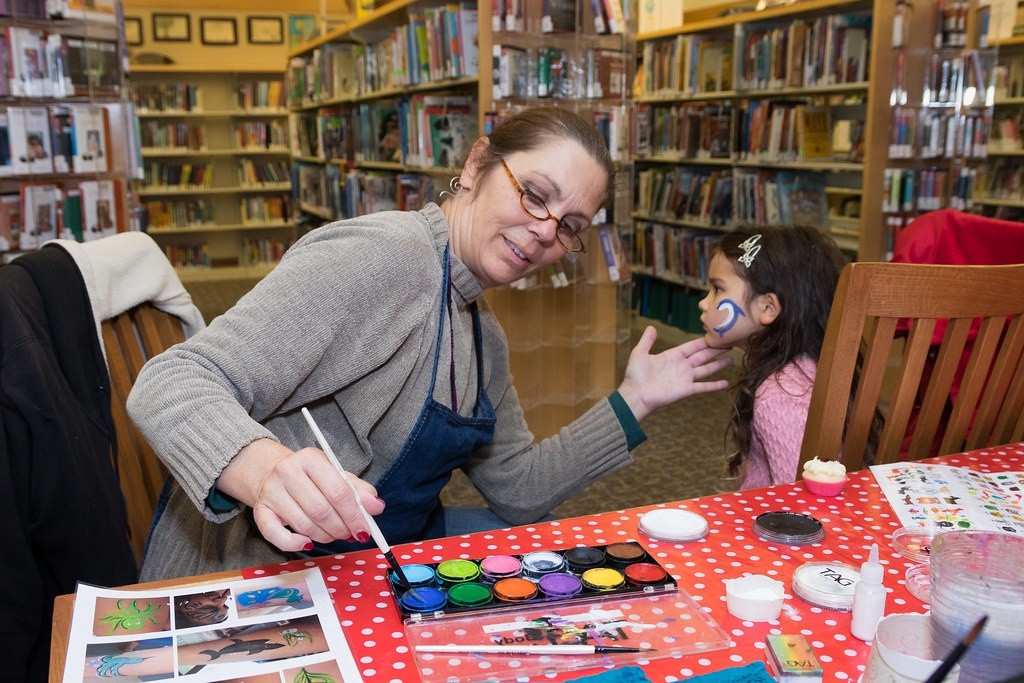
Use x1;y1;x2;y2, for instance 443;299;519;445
851;542;886;641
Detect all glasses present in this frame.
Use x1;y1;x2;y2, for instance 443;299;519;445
489;137;588;254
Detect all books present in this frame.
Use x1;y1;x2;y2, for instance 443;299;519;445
0;0;631;291
630;0;1024;286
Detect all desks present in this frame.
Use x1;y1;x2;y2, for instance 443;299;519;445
51;435;1024;683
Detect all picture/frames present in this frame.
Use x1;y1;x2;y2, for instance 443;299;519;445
288;13;316;50
153;12;191;42
248;15;284;45
123;17;143;46
200;17;238;46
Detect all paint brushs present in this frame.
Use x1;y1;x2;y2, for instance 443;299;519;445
302;402;428;604
920;614;991;683
416;640;659;656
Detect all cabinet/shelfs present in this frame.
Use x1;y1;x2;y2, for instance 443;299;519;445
0;0;1024;440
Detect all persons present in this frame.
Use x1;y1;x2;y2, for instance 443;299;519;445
697;224;886;494
125;106;733;583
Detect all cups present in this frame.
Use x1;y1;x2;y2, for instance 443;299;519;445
930;531;1024;683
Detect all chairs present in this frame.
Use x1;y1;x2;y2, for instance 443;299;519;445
795;262;1024;487
0;231;186;576
